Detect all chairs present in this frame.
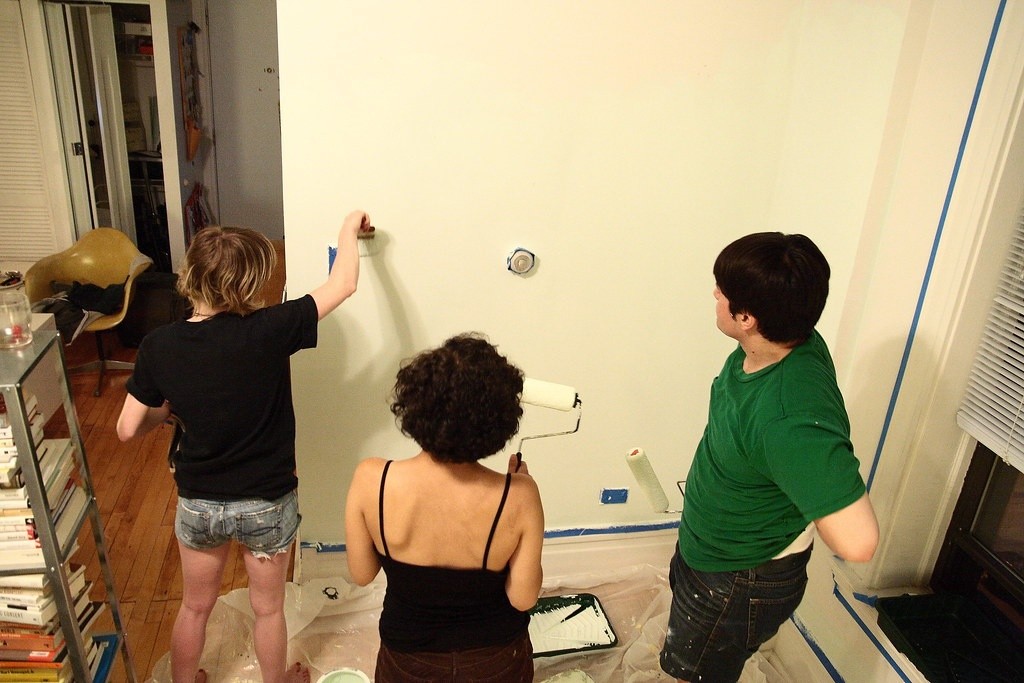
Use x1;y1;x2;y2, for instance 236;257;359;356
25;227;154;396
127;150;170;261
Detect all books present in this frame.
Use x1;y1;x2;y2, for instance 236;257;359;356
0;397;116;682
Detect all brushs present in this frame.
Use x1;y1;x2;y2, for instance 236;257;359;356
357;217;379;257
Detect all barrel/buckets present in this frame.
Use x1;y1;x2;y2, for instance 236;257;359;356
316;668;371;683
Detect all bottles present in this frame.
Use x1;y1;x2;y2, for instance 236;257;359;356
0;270;33;349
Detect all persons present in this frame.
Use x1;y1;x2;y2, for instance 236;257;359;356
118;211;370;683
657;230;879;683
346;330;544;683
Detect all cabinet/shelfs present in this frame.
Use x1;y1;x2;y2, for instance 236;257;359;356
0;331;138;683
